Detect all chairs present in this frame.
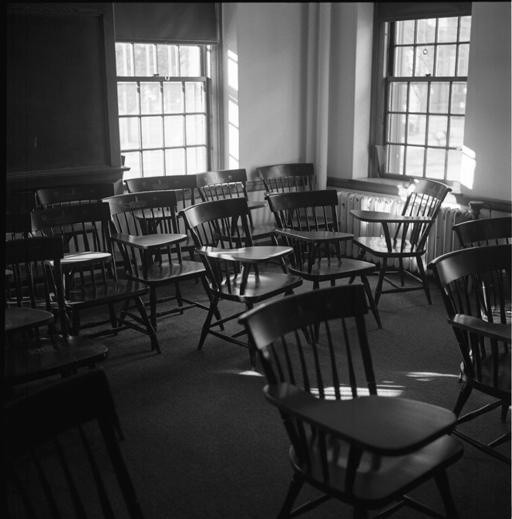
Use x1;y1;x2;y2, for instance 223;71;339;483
129;175;211;263
0;369;142;519
34;184;118;211
0;237;109;381
240;284;464;519
100;188;223;332
430;243;512;464
345;176;452;303
26;202;164;354
456;214;512;245
177;197;320;371
257;163;339;271
193;168;288;276
267;190;382;328
